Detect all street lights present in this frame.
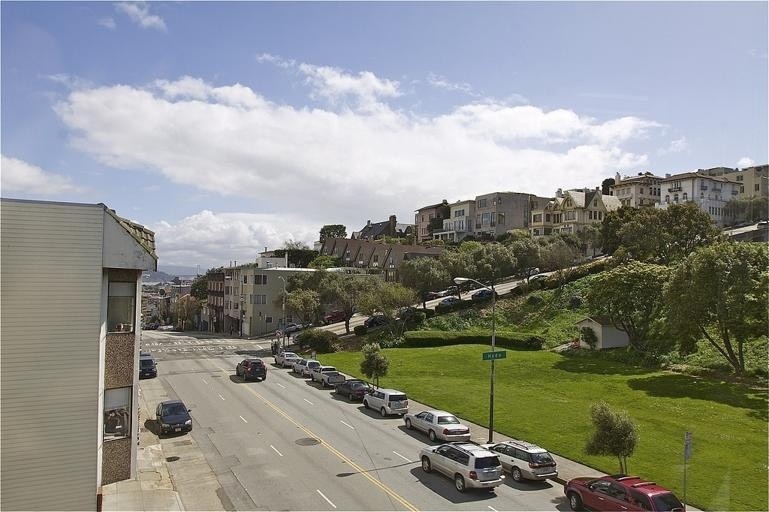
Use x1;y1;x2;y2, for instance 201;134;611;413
277;275;286;348
454;277;496;440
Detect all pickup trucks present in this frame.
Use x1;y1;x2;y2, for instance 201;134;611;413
571;252;593;264
310;365;347;389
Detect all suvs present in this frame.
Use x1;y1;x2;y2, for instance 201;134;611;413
419;442;505;492
564;474;686;512
236;359;268;381
478;441;558;481
139;356;157;379
363;388;408;417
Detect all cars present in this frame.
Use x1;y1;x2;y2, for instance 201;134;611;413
334;379;376;401
424;278;496;315
403;410;471;442
156;399;192;436
285;309;347;333
364;315;394;327
513;265;540;278
402;306;434;323
274;349;322;377
